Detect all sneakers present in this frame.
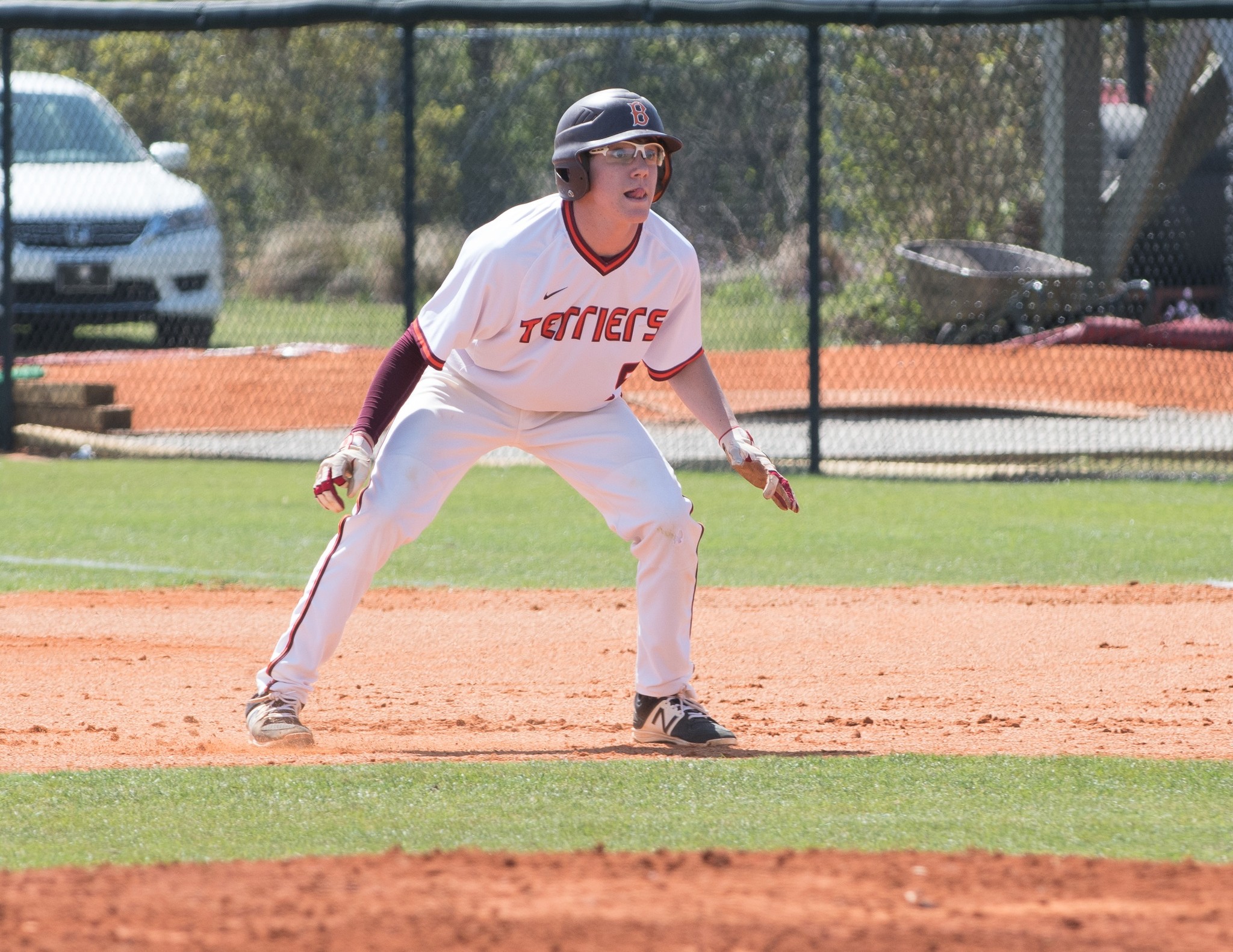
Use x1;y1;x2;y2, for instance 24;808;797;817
246;692;314;747
631;692;736;746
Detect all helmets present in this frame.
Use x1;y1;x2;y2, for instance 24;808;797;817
552;89;683;203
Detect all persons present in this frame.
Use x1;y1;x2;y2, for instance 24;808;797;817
244;88;799;748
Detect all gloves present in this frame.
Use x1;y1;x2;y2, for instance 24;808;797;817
313;432;374;513
719;427;800;513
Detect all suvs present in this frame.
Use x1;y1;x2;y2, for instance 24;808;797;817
0;71;224;348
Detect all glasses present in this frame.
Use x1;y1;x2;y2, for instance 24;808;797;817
587;140;665;166
